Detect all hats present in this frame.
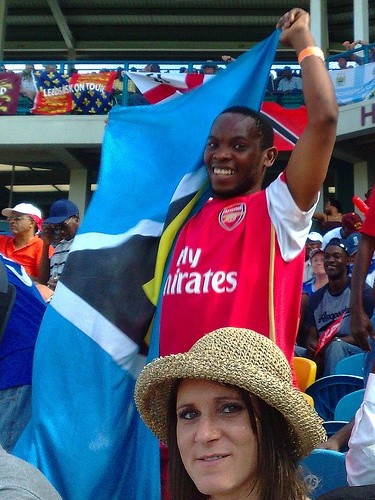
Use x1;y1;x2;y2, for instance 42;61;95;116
202;60;218;67
340;213;362;231
325;237;350;254
307;232;323;242
347;232;361;255
44;199;79;223
2;203;41;223
309;247;325;257
134;326;327;455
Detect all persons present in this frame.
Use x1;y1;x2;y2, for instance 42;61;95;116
0;63;160;105
156;7;340;500
294;184;375;500
0;443;64;500
266;64;303;91
179;54;237;74
0;253;47;453
0;199;82;306
133;327;326;500
337;39;375;69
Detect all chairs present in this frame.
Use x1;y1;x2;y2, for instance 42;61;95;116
297;374;365;500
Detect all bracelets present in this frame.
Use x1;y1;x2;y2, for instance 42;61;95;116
297;46;325;64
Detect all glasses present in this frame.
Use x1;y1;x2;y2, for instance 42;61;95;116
6;216;32;222
51;217;73;228
201;66;213;69
